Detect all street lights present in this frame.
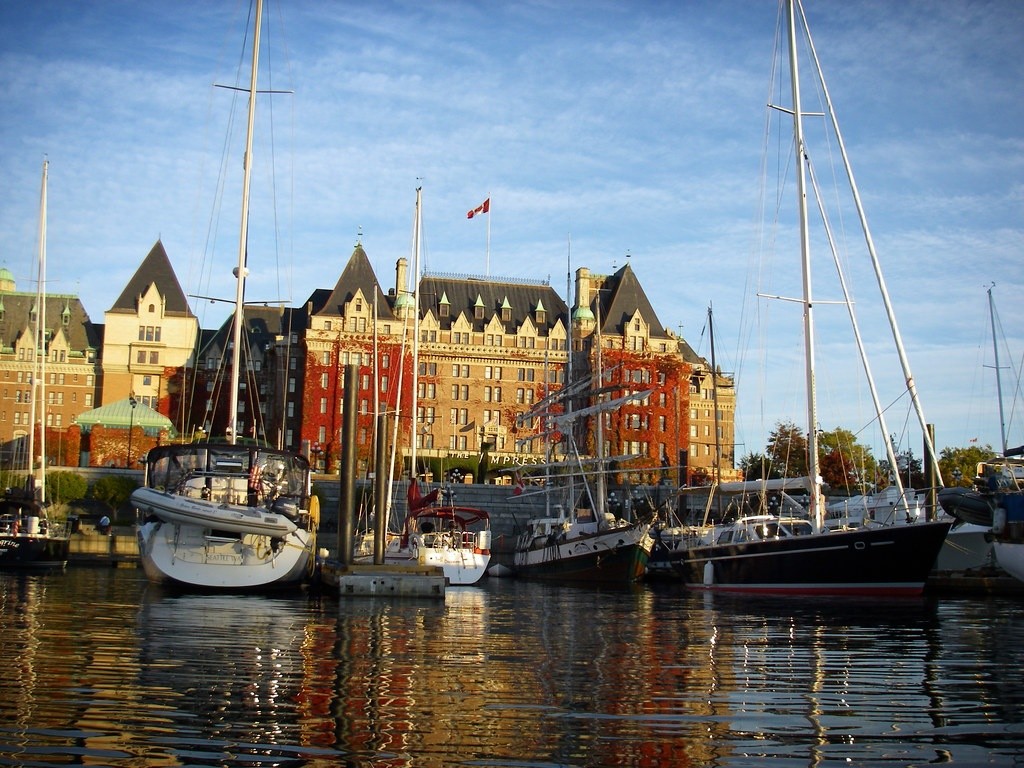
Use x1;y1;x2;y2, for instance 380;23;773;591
126;394;138;470
310;442;323;472
952;466;963;488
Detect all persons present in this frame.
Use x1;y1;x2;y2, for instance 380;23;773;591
100;514;110;535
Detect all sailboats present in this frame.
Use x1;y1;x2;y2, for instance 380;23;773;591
130;0;321;591
0;150;74;568
352;176;493;586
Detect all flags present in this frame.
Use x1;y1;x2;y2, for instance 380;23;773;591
13;513;18;539
249;460;261;496
466;199;488;218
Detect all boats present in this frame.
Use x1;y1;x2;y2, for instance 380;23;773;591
487;564;512;578
498;231;688;584
646;2;1024;609
